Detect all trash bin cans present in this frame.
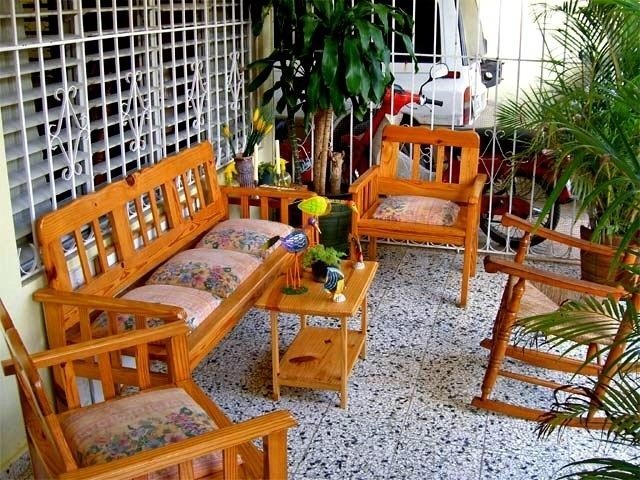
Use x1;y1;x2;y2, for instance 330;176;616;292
319;194;353;257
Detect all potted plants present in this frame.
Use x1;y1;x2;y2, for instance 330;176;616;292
301;244;348;283
244;0;419;259
494;0;640;287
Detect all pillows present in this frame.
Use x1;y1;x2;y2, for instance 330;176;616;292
60;388;243;480
90;218;296;332
372;194;461;227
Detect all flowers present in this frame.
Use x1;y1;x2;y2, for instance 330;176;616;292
222;107;274;157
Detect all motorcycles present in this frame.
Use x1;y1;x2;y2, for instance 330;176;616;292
274;63;577;249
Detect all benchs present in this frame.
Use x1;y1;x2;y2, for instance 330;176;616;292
31;140;319;412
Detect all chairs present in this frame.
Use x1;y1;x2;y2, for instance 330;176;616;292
471;212;640;429
0;299;300;480
348;124;488;308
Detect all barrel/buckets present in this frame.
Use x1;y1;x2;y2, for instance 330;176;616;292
578;223;640;286
318;202;353;258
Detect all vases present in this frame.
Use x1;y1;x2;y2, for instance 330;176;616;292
234;155;255;189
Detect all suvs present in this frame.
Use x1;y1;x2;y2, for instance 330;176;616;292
271;1;503;130
